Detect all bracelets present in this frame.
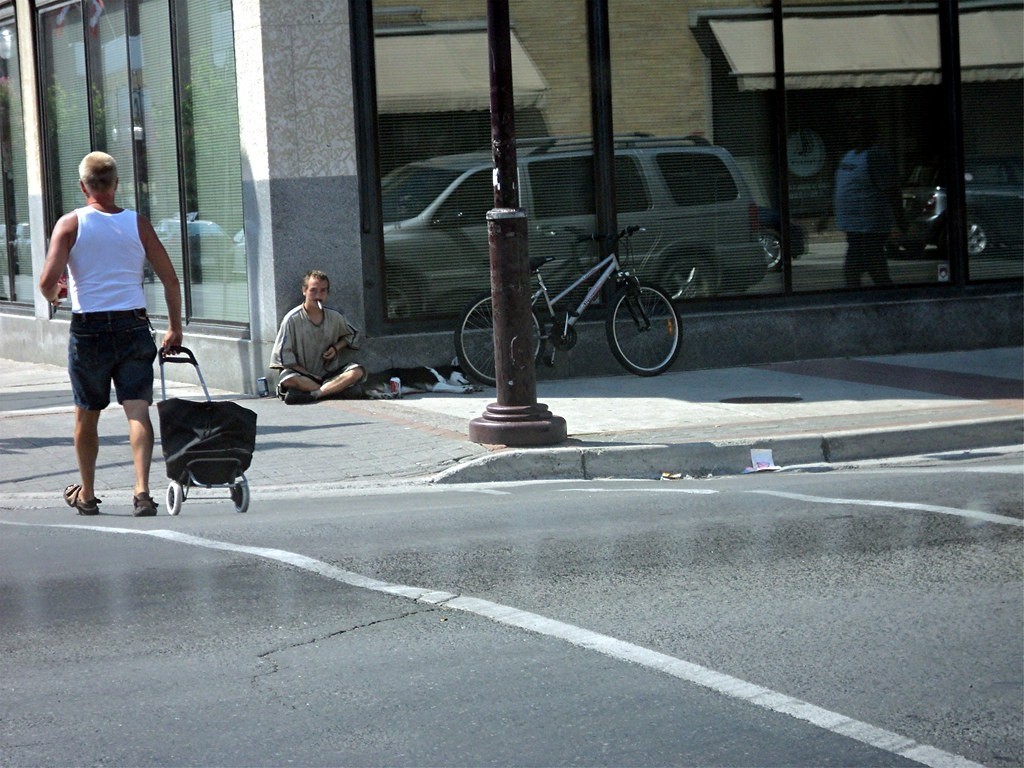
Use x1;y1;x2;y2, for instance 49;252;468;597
330;344;340;355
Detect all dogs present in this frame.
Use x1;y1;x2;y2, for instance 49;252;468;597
339;357;485;399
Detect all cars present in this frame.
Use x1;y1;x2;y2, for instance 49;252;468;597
157;211;236;283
888;150;1024;258
759;206;807;273
146;225;174;285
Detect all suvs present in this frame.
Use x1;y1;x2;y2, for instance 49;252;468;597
382;130;766;319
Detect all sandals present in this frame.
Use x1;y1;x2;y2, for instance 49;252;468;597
64;484;102;514
133;492;159;516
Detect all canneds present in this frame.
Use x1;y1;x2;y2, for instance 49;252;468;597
57;271;67;302
390;377;401;398
257;377;269;397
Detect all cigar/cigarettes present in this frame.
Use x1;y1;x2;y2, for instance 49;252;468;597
317;300;323;310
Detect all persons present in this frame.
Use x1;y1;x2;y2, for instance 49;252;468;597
38;150;182;518
268;268;365;405
830;121;908;301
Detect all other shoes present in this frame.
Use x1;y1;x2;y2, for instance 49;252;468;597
285;389;314;404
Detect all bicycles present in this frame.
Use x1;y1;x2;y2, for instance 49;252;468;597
453;226;684;389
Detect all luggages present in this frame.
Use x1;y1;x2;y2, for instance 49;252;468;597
158;345;257;516
